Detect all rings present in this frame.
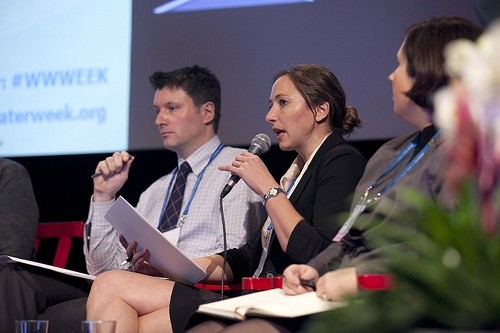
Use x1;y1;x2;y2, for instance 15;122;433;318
322;294;329;300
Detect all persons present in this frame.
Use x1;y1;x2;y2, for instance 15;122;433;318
85;64;359;333
184;15;489;333
0;65;265;333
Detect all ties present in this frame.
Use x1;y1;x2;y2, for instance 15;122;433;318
158;161;192;233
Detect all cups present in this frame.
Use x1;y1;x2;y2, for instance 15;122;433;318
15;320;49;333
81;321;117;333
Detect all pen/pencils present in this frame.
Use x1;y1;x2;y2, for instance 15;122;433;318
91;148;137;177
121;256;131;266
300;278;316;287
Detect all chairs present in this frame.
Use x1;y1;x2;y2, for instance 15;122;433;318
33;221;412;292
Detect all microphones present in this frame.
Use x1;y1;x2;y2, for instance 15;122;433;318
220;133;271;198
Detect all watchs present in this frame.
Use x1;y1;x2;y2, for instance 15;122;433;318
262;186;286;207
